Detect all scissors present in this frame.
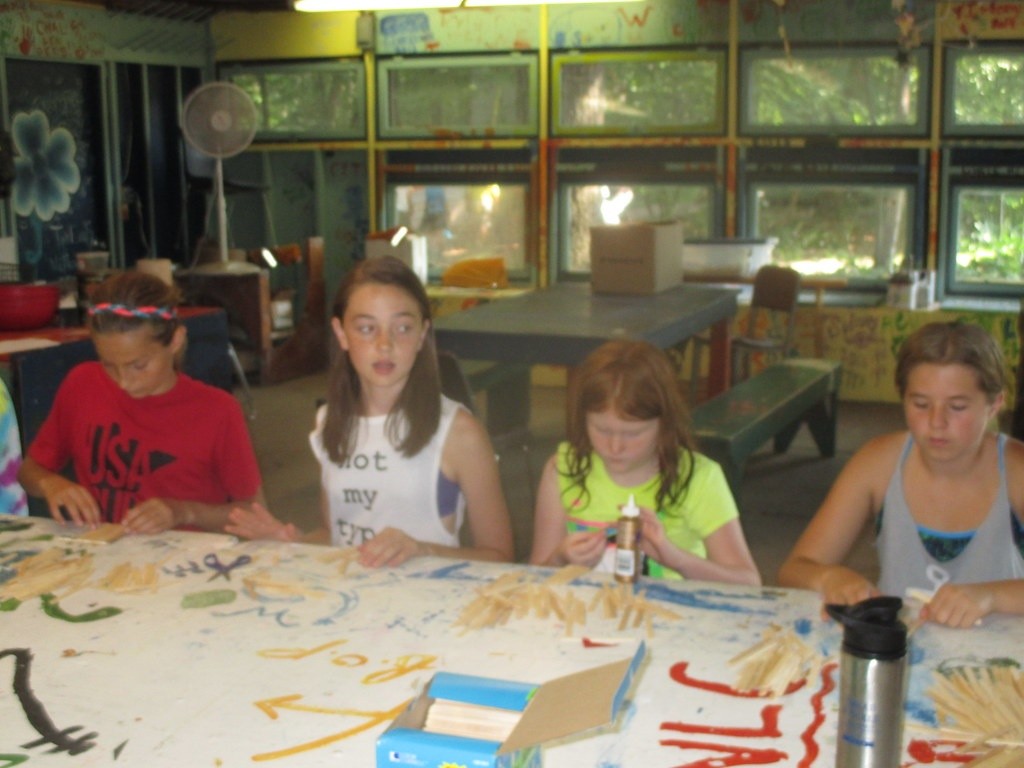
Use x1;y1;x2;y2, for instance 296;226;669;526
203;553;251;583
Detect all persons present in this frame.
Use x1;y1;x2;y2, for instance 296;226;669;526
225;254;514;571
0;369;28;516
17;270;269;541
777;323;1024;630
529;339;763;589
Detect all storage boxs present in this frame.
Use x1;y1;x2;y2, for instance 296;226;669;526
375;641;645;768
365;225;427;284
589;220;685;294
682;236;779;274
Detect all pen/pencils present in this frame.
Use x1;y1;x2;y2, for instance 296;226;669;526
905;587;983;626
582;637;631;648
907;615;930;639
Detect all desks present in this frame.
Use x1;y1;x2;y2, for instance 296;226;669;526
0;305;255;463
1;511;1024;768
432;282;754;400
423;286;1024;413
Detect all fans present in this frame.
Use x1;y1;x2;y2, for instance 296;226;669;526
181;81;260;276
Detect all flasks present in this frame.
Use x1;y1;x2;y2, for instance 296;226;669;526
824;596;909;768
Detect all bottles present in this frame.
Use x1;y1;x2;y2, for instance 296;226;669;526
614;493;642;584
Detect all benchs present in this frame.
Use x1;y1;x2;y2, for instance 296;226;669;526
692;358;843;502
316;360;532;436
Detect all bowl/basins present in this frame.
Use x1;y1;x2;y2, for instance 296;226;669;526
77;251;109;275
0;284;62;330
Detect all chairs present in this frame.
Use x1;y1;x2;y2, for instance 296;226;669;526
691;264;800;397
